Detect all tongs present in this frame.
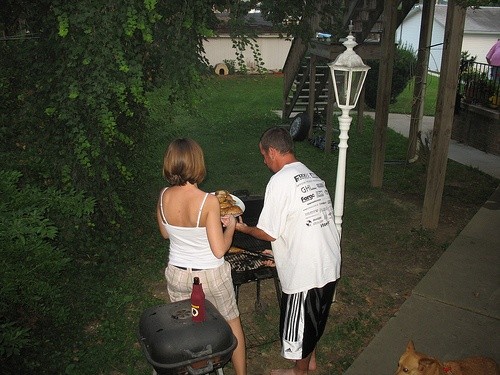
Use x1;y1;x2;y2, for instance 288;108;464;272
230;247;274;263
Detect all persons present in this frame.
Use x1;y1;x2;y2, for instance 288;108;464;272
157;136;246;375
223;127;341;375
486;39;500;80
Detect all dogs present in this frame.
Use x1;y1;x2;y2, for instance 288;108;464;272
394;340;500;375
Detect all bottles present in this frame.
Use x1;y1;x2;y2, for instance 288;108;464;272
190;277;205;323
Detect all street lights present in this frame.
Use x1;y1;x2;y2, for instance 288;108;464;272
325;19;371;306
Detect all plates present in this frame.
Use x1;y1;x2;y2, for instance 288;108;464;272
210;192;245;218
